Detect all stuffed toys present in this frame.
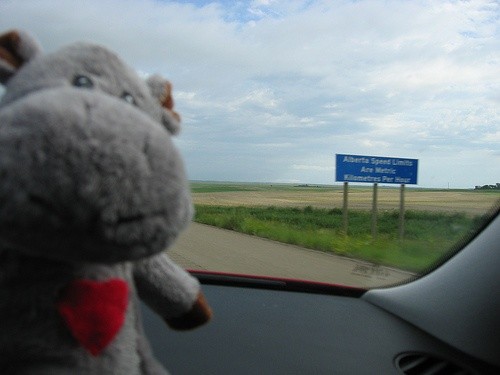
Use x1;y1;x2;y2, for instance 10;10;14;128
0;27;213;375
